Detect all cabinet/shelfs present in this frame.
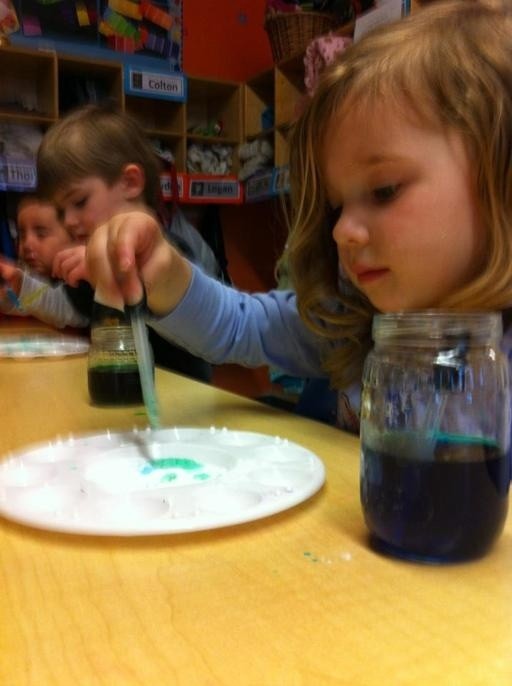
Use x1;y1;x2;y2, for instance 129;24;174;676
241;0;439;171
0;43;243;175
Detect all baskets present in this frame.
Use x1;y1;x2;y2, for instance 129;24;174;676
263;5;333;66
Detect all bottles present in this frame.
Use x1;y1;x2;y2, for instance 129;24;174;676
22;274;67;310
1;274;24;315
125;278;163;432
358;310;511;567
87;324;155;408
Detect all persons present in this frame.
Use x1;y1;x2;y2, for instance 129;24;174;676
0;100;218;384
85;0;512;476
1;192;74;282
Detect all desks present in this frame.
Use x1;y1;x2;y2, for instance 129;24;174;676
1;310;512;684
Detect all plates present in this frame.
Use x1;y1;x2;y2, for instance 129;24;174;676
0;332;91;364
0;421;329;542
